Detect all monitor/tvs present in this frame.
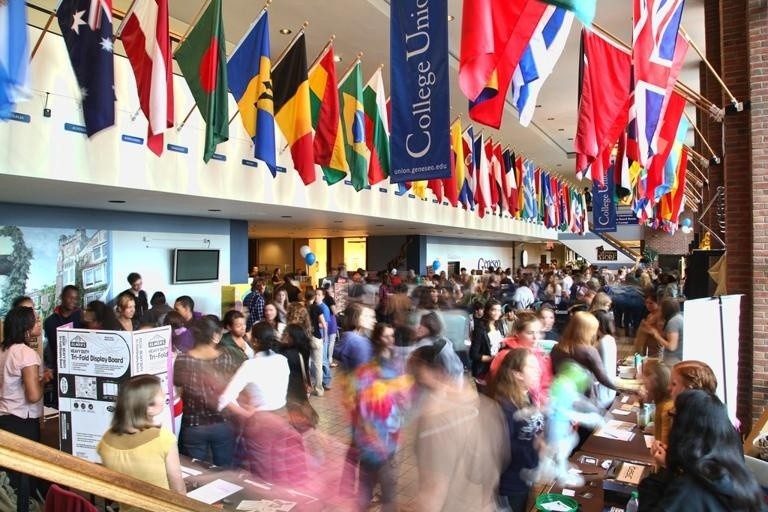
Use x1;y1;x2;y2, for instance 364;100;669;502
172;247;222;285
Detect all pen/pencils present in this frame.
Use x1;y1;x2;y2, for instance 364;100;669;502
578;472;599;475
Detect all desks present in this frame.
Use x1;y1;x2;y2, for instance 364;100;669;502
528;392;667;512
177;453;332;511
39;408;59;449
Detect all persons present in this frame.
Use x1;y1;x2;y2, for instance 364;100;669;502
1;258;767;512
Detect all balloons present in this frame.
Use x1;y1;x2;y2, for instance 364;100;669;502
305;253;316;265
300;246;311;259
682;217;693;233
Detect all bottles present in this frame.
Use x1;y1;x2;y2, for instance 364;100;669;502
636;402;647;430
626;491;639;512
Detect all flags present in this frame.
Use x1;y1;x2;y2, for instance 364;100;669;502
0;0;687;238
